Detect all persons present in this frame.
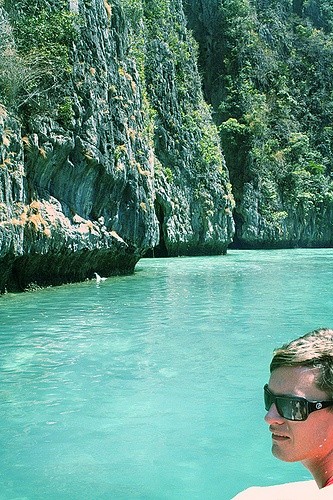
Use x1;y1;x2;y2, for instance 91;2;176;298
229;327;333;500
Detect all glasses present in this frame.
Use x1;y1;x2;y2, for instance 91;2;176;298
264;384;332;421
